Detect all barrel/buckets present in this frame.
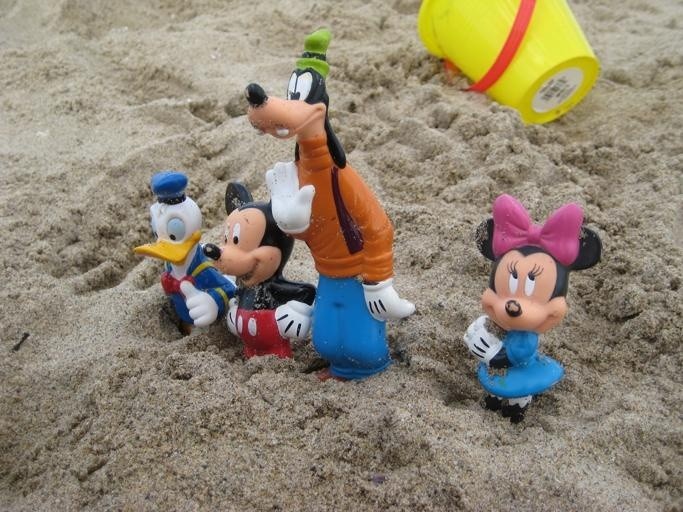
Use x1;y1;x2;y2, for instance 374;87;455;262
416;0;601;128
